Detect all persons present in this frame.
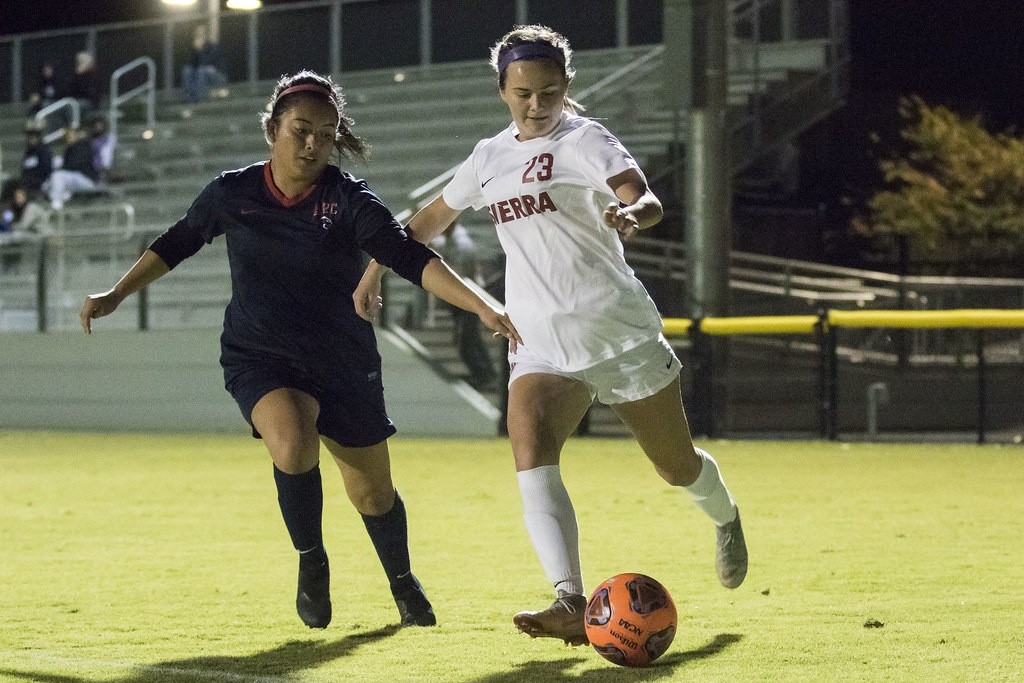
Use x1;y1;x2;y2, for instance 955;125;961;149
0;52;115;234
433;220;496;384
353;26;748;645
181;24;227;103
79;72;523;629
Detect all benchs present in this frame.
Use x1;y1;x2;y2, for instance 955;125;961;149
0;40;829;335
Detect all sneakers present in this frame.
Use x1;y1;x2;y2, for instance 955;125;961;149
390;570;436;626
513;589;590;647
715;504;748;589
296;549;332;629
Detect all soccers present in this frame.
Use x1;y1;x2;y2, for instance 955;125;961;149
583;573;677;668
